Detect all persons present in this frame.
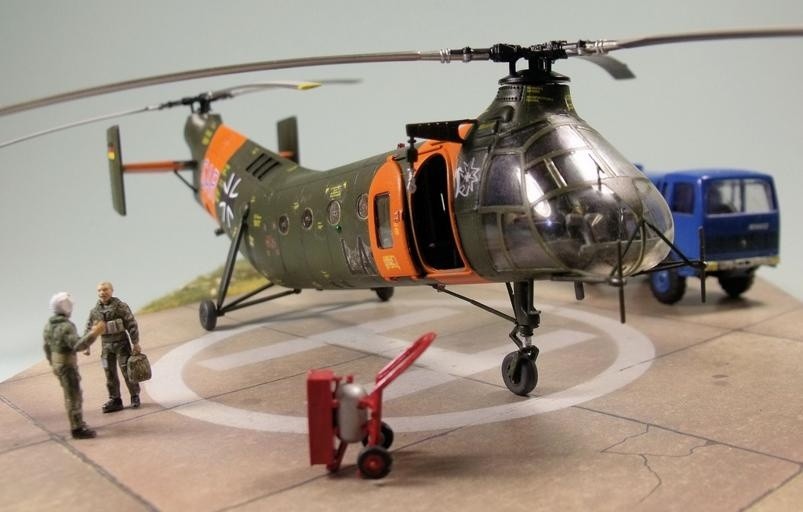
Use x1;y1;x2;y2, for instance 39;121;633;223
80;281;151;413
42;291;105;440
